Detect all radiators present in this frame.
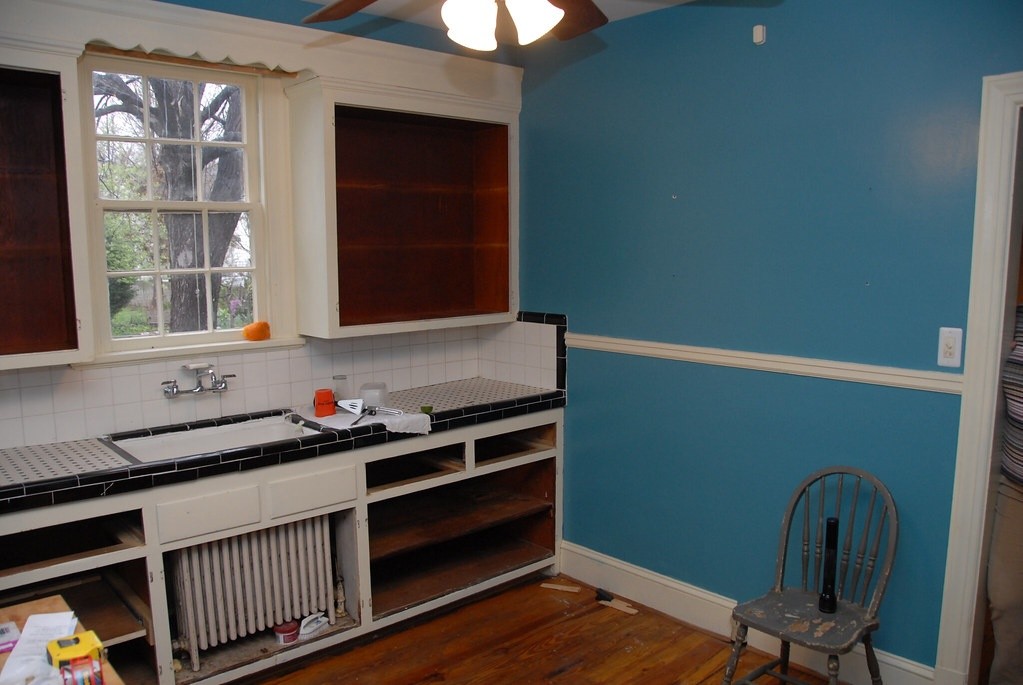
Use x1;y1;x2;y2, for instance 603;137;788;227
171;512;336;673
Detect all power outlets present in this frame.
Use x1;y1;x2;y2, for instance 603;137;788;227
937;327;963;368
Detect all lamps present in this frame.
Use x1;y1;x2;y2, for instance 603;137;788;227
441;0;566;52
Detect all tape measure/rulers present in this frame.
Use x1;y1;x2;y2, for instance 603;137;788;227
46;629;108;668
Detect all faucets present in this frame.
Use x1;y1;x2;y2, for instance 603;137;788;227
197;372;217;388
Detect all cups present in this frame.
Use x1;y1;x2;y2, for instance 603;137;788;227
314;389;336;417
332;375;353;401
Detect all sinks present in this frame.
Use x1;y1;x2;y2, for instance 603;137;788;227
97;407;339;467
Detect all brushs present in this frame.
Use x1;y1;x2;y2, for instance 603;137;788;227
298;612;330;641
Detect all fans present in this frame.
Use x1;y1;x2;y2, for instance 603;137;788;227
302;0;777;40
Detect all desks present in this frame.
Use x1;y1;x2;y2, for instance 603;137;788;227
0;595;125;685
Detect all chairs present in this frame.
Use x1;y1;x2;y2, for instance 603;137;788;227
721;465;899;685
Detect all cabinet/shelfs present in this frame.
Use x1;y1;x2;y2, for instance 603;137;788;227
0;407;566;685
285;75;516;340
0;34;94;371
